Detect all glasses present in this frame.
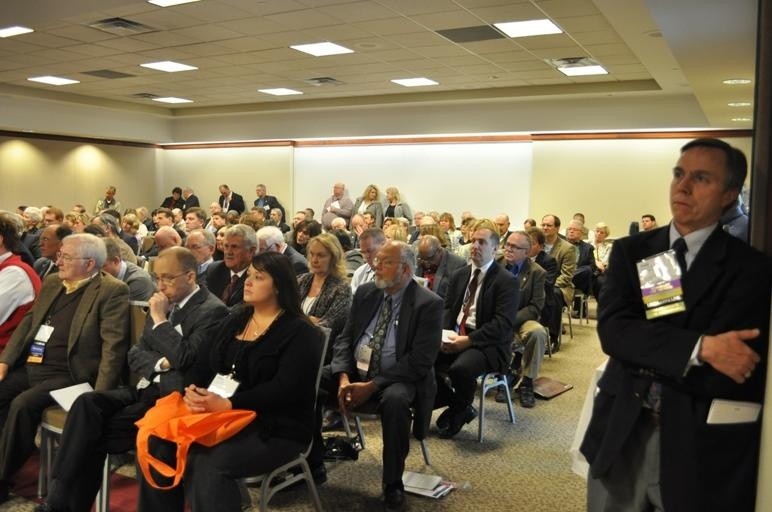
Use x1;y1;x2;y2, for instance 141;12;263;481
371;257;405;268
153;271;190;285
504;244;525;250
265;243;274;251
56;250;89;262
417;250;439;260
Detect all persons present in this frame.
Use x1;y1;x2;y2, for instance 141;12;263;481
2;183;612;512
581;135;772;511
642;215;659;233
720;192;751;245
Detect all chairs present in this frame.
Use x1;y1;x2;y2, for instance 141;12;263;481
559;306;576;348
474;351;515;444
573;288;589;326
323;405;430;469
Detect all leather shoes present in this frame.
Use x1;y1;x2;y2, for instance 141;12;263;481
545;340;559;354
383;481;406;511
436;408;452;429
518;381;535;407
495;373;520;401
269;465;329;505
437;405;478;440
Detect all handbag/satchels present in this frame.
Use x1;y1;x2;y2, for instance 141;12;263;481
133;390;256;491
325;433;361;462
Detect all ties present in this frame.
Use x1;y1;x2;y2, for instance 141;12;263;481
671;238;690;272
221;275;238;304
459;269;481;335
366;296;393;380
225;197;227;209
